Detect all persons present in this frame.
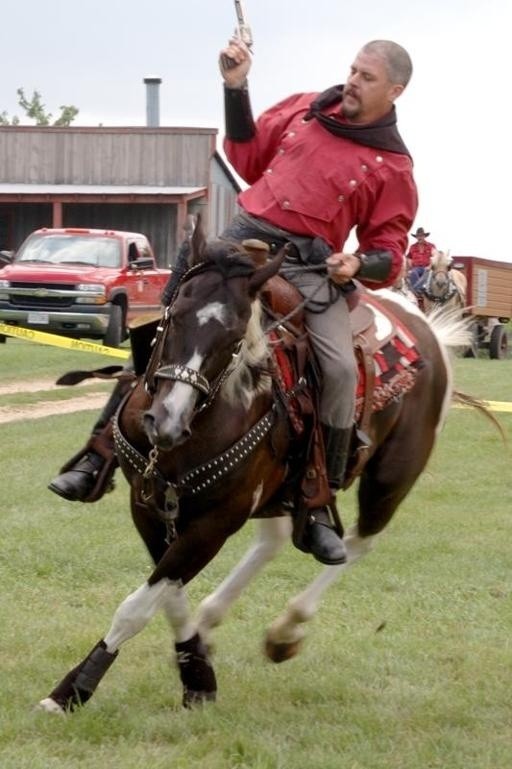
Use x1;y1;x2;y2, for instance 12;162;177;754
406;227;437;313
48;20;420;566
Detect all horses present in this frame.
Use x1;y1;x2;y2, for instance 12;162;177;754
423;247;467;318
38;213;507;724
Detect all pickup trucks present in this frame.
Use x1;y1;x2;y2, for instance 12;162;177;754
0;222;173;349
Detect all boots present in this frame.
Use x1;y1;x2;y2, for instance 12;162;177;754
46;375;139;500
290;418;354;566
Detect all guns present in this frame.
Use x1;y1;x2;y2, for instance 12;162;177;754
221;0;254;68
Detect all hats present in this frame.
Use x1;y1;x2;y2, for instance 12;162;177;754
410;227;431;238
